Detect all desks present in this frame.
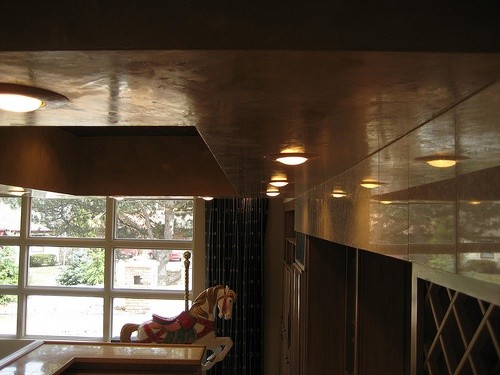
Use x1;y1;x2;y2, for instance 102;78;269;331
0;340;208;374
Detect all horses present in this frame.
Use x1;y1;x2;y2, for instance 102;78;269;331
120;285;237;375
